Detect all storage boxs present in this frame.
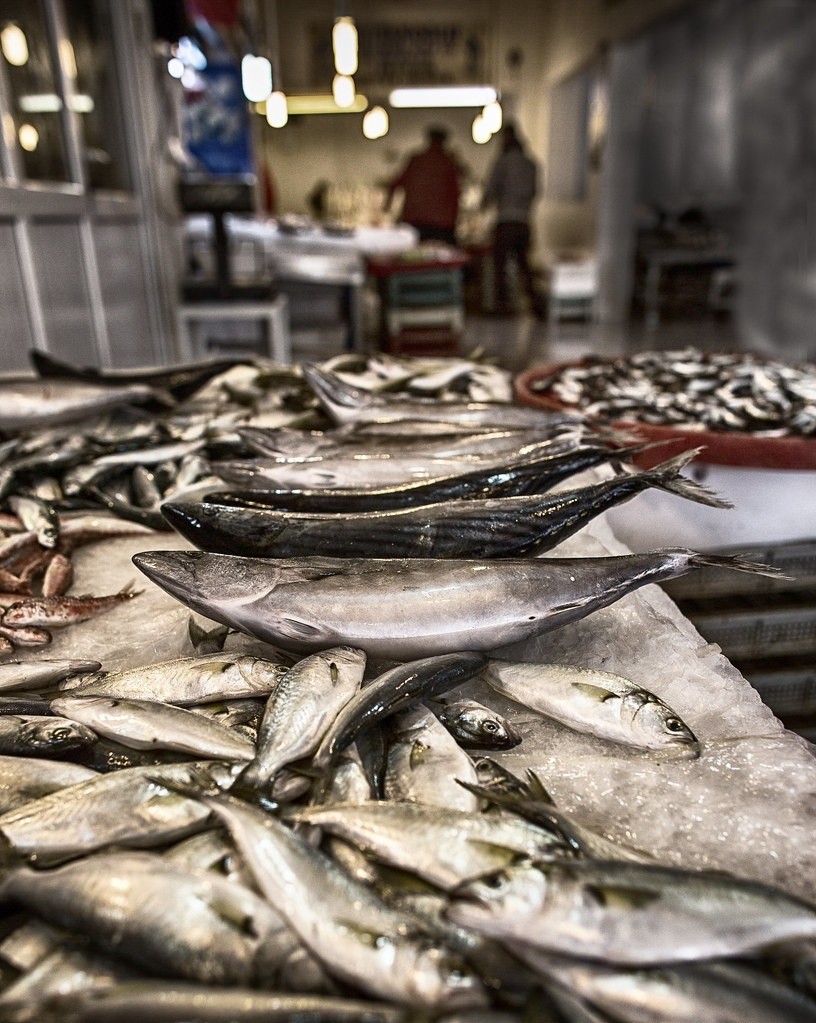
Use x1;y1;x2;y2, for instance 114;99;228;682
379;270;467;359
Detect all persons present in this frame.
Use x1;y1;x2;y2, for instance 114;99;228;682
380;122;466;248
477;126;547;321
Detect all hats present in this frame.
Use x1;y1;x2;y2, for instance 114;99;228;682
424;120;452;138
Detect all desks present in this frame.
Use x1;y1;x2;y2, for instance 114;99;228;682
637;244;735;349
268;280;362;358
174;294;291;365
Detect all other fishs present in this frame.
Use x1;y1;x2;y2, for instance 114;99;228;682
0;348;816;1023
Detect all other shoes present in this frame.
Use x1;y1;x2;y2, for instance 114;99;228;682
532;298;548;321
488;302;514;318
